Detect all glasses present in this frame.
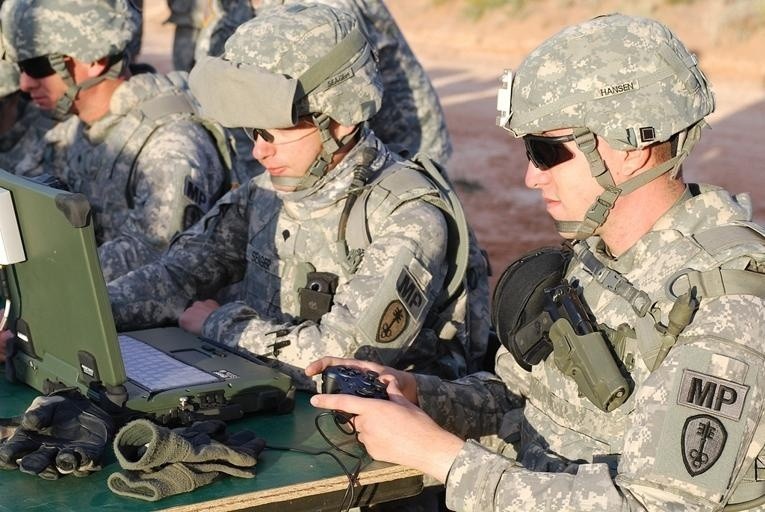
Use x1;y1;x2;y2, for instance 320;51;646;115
16;58;56;78
243;128;318;145
522;136;575;170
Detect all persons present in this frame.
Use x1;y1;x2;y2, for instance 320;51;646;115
301;13;765;512
1;1;491;391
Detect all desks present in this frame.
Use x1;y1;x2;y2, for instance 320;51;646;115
0;358;424;510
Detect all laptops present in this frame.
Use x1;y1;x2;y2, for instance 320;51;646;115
0;167;297;438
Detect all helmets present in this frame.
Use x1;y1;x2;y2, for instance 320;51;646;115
223;1;385;128
1;0;143;63
497;12;714;145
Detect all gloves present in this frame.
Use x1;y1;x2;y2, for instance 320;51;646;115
108;419;257;501
0;387;109;480
